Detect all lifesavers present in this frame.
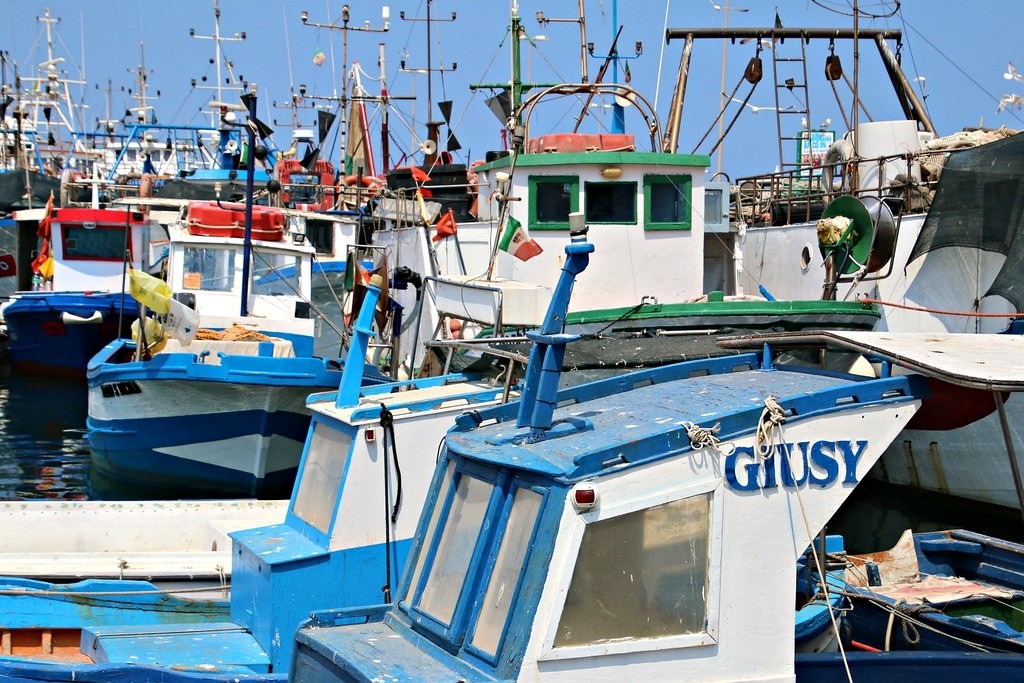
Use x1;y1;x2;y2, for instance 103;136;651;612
336;176;378;211
139;172;152;215
821;139;854;202
33;166;57;178
57;169;73;207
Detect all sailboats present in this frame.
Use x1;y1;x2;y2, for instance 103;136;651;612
0;0;485;301
659;0;1024;542
436;0;882;388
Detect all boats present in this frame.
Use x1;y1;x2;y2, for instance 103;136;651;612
83;84;387;503
0;277;519;682
0;154;154;387
287;216;1024;683
